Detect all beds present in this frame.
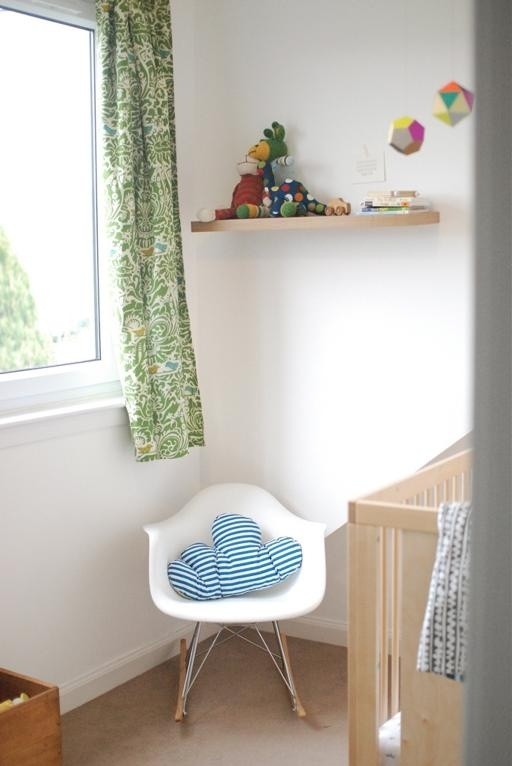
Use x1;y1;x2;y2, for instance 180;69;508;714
347;450;471;766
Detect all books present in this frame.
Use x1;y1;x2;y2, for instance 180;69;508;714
354;189;431;216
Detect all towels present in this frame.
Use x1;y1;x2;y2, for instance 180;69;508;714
416;502;471;682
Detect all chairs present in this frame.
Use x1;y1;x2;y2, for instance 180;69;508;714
144;484;328;724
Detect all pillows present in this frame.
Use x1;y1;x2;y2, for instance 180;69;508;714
168;514;303;600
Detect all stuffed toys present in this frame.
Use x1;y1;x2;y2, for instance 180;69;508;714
193;152;271;222
261;178;326;217
245;118;294;200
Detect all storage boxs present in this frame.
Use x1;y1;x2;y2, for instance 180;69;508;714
0;668;63;766
